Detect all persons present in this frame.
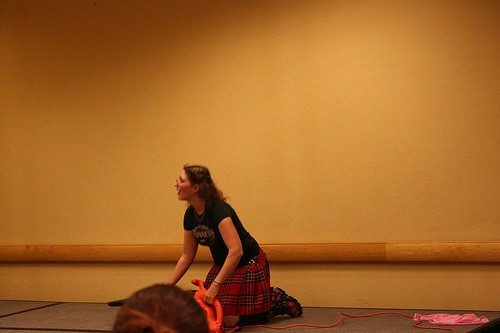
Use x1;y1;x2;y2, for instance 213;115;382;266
110;283;210;333
164;163;304;329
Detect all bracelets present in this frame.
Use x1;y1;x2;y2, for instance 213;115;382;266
213;280;221;286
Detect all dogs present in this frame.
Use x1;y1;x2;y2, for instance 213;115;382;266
107;283;209;333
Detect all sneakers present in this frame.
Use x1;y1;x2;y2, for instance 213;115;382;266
270;286;304;318
